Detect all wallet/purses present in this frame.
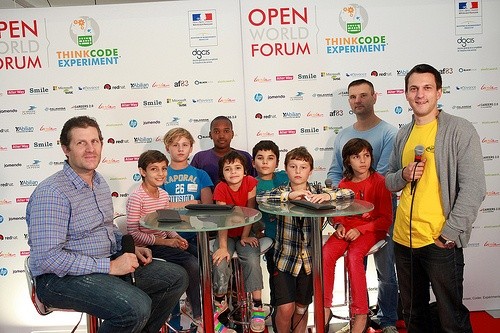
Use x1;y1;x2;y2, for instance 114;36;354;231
157;209;183;223
184;204;235;211
291;200;336;211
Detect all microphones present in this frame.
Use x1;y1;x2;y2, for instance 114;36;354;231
325;179;332;188
411;145;424;195
122;235;136;284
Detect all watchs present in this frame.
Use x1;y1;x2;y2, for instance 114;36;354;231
438;235;455;250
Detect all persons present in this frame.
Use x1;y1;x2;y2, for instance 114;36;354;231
26;116;189;333
322;138;393;333
190;116;258;320
385;63;486;333
212;151;265;333
325;79;398;333
126;150;234;333
251;140;354;333
159;128;214;333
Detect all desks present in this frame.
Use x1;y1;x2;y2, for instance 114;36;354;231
259;199;374;333
140;205;262;333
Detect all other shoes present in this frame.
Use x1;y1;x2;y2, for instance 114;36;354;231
351;312;368;333
213;295;228;323
250;303;267;332
197;315;237;333
180;297;200;326
382;326;399;333
324;307;333;327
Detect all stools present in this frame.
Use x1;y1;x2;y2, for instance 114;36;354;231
328;233;391;333
25;257;102;333
208;237;274;333
